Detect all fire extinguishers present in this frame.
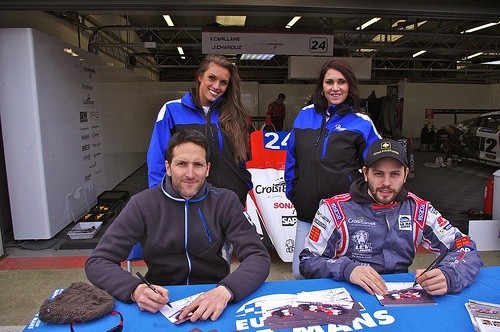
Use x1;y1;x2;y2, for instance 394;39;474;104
483;175;494;216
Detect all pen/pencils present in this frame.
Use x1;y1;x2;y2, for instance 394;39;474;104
136;272;173;308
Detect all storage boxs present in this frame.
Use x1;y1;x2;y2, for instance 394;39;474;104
68;220;103;240
97;190;129;202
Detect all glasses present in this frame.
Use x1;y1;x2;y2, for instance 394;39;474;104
70;311;124;332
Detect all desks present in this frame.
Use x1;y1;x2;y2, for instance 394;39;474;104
24;265;500;332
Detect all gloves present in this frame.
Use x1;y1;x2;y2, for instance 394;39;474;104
265;118;272;125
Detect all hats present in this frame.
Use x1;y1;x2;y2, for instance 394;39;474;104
365;138;408;171
39;281;114;325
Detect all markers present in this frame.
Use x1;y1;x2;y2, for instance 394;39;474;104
413;253;447;286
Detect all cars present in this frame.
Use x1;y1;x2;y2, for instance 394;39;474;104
435;111;500;168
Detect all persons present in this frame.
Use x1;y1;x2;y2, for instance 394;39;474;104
284;58;385;280
299;139;483;294
265;93;286;132
84;129;270;322
147;54;252;269
